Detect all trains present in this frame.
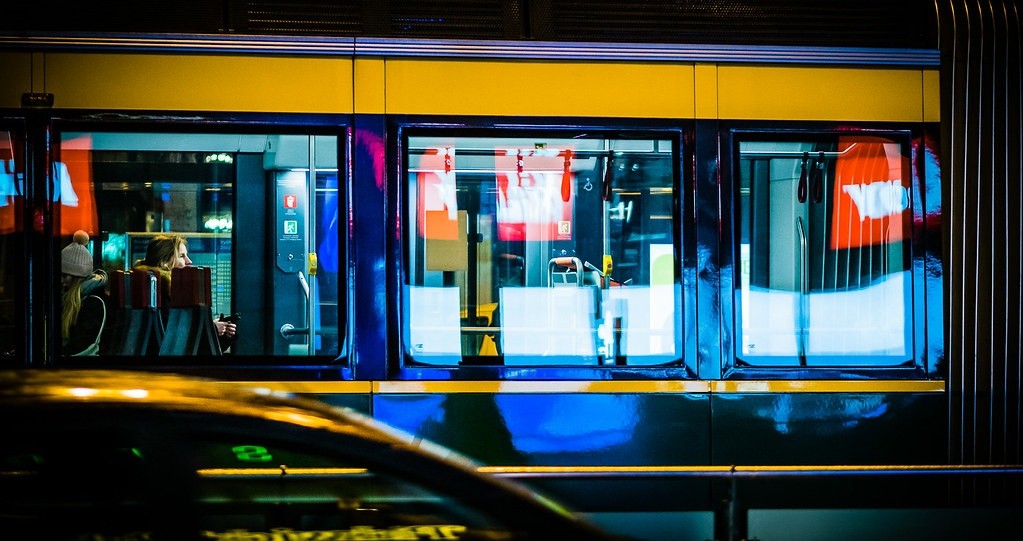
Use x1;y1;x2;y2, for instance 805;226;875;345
0;27;947;383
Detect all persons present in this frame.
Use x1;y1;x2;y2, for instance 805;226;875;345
135;235;237;353
60;231;112;357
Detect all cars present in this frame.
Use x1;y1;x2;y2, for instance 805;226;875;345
0;369;622;541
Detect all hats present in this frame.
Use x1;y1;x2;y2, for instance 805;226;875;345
61;230;92;277
